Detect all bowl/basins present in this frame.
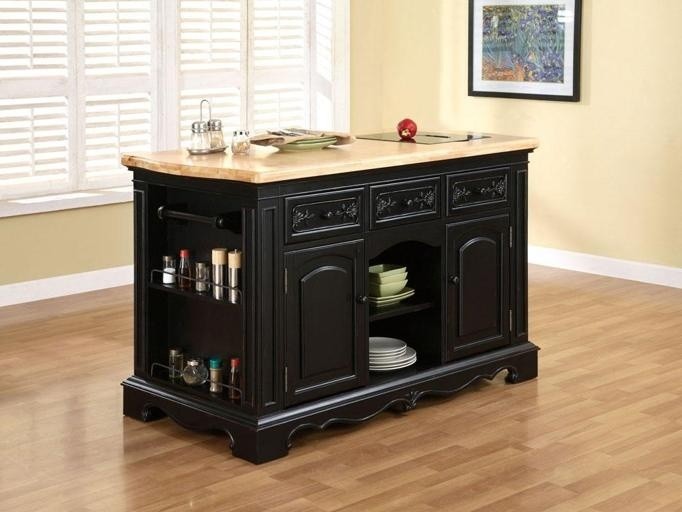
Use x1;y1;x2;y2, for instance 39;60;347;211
367;262;410;298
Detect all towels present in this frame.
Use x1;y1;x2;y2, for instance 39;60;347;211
248;128;356;146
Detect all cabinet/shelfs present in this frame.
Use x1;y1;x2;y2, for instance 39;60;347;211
283;171;368;458
133;167;281;419
368;151;511;418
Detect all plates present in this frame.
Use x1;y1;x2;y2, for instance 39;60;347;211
368;336;420;372
367;287;417;309
273;137;337;152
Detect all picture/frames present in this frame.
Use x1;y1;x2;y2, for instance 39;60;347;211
467;0;583;101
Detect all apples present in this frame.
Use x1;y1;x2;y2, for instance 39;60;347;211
396;118;417;139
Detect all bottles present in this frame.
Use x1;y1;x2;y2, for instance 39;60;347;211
190;121;208;149
231;130;250;157
207;118;223;150
168;347;243;401
162;246;242;304
